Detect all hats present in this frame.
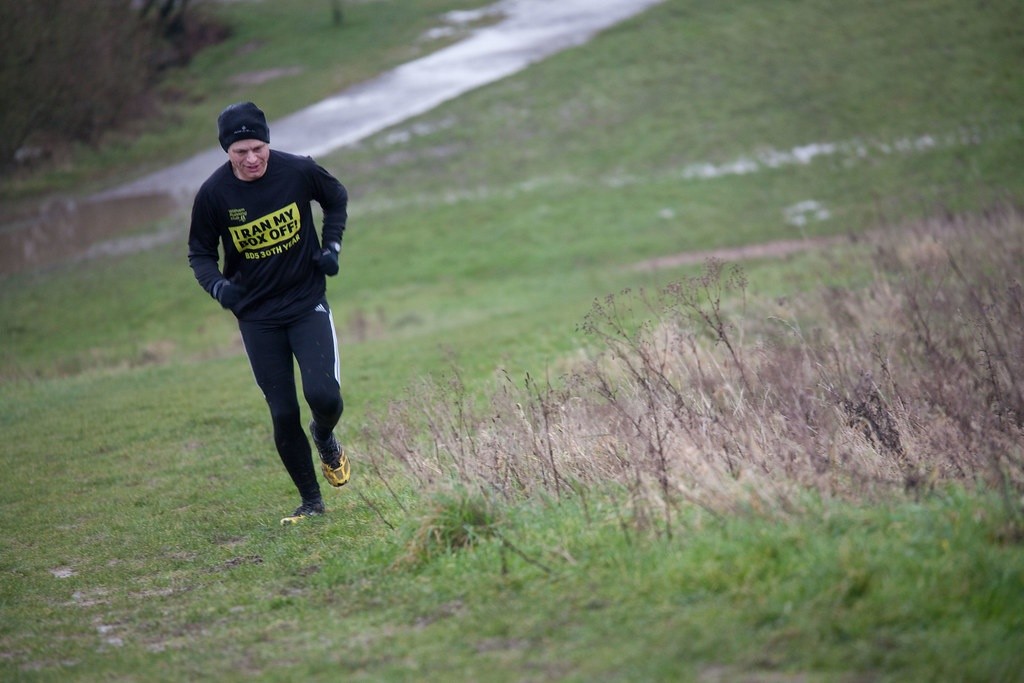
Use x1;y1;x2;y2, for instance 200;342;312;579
218;102;270;152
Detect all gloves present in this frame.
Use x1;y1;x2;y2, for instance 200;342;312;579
218;281;247;311
316;247;340;275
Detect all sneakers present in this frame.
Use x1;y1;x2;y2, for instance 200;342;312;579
309;420;352;486
278;503;324;526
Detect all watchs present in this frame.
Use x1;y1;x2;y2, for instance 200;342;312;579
324;241;341;253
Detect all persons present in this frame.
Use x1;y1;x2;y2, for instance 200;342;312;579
188;100;352;525
